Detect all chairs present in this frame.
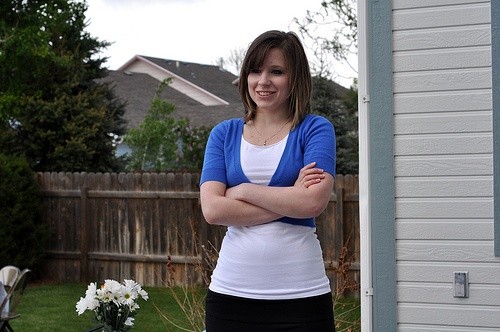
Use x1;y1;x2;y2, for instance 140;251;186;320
0;265;20;304
0;268;31;332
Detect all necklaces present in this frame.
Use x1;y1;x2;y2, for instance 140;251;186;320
252;115;292;147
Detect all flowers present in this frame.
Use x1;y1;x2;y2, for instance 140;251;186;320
75;279;150;327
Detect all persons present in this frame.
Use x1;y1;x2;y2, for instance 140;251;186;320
197;31;338;331
